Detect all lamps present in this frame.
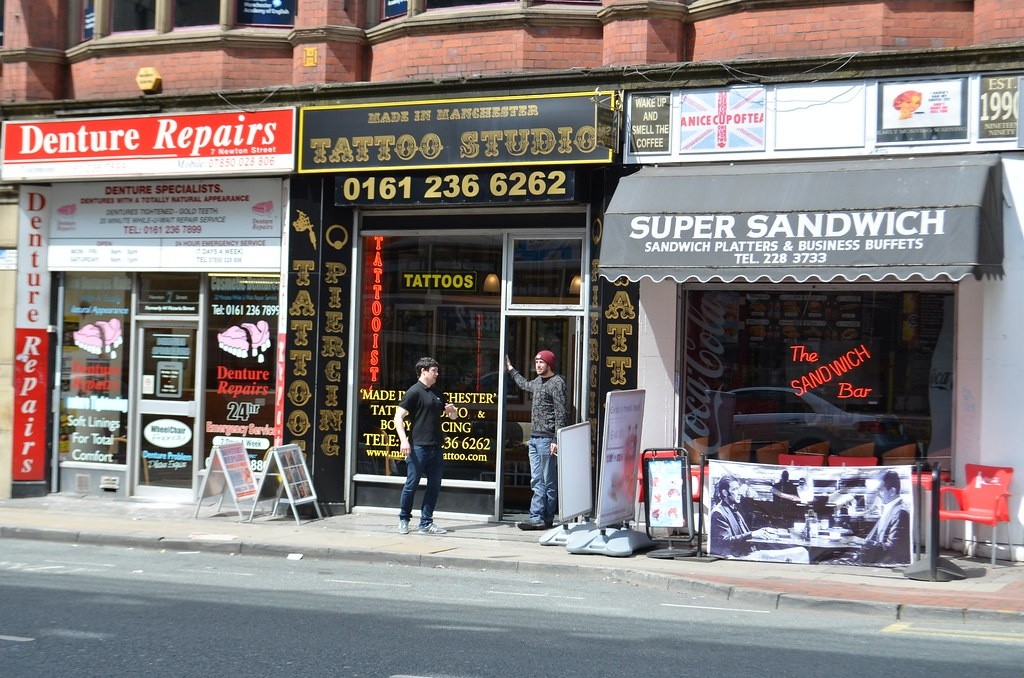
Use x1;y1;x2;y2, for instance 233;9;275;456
483;235;500;293
569;273;580;295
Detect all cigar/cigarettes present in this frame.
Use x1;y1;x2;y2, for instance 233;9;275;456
553;453;558;456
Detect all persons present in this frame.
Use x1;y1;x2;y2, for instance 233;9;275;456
504;350;568;531
770;471;801;511
797;476;815;502
393;356;457;534
828;482;855;511
710;474;774;556
842;470;910;565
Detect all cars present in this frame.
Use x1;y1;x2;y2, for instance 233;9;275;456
682;384;914;469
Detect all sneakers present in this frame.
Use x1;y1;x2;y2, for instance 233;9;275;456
419;523;446;534
399;520;409;533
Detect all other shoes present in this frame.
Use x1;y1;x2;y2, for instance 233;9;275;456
544;520;553;528
517;522;546;530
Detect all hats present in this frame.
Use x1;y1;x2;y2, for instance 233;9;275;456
534;350;555;369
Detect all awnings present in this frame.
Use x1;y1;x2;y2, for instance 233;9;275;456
599;154;1006;283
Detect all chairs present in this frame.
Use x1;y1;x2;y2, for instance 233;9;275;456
827;454;878;467
634;449;702;535
778;454;824;466
938;463;1015;569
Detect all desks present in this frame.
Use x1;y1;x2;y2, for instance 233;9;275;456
911;470;951;561
